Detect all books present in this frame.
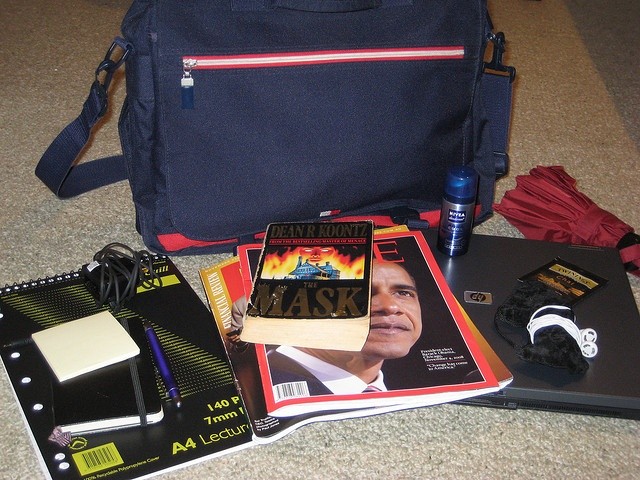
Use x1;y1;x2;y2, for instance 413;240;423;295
1;256;252;476
31;310;164;437
240;221;374;354
235;230;501;417
198;221;510;444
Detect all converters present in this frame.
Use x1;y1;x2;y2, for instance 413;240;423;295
81;258;140;298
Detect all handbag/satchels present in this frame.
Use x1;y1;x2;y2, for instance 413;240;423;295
35;0;516;256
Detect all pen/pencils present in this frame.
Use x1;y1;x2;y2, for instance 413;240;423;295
145;320;183;409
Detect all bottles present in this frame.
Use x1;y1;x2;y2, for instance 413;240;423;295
437;166;479;257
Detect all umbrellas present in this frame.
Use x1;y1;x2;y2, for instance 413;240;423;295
494;165;638;272
